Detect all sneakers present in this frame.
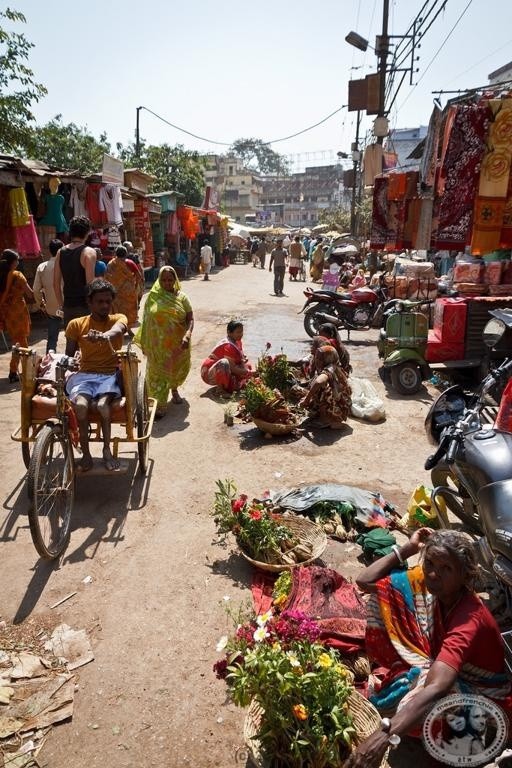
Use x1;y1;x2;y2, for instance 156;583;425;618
173;391;182;404
215;390;228;396
156;407;167;420
10;374;19;382
279;291;283;295
275;291;280;295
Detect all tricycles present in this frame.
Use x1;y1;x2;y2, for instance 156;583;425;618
11;335;157;560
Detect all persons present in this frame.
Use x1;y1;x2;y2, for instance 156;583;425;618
301;234;313;259
290;335;335;401
310;243;326;282
121;240;145;309
200;321;259;399
223;241;239;266
342;528;510;667
60;277;128;469
269;239;289;296
88;247;106;282
32;239;64;357
199;239;213;281
315;233;326;250
106;246;141;328
247;236;254;262
53;215;95;356
286;236;307;282
251;240;260;266
256;236;270;269
283;234;293;263
133;264;194;419
298;346;353;431
0;247;34;384
286;322;352;379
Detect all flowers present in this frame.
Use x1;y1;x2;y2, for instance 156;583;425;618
259;343;290;389
236;377;285;418
272;566;295;613
210;478;313;569
212;582;364;767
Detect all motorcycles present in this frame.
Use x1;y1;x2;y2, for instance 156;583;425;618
424;356;512;593
297;268;393;340
377;297;433;396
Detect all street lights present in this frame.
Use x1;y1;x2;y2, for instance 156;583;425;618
345;1;398;169
336;111;361;234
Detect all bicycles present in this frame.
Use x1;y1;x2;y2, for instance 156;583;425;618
298;257;308;282
